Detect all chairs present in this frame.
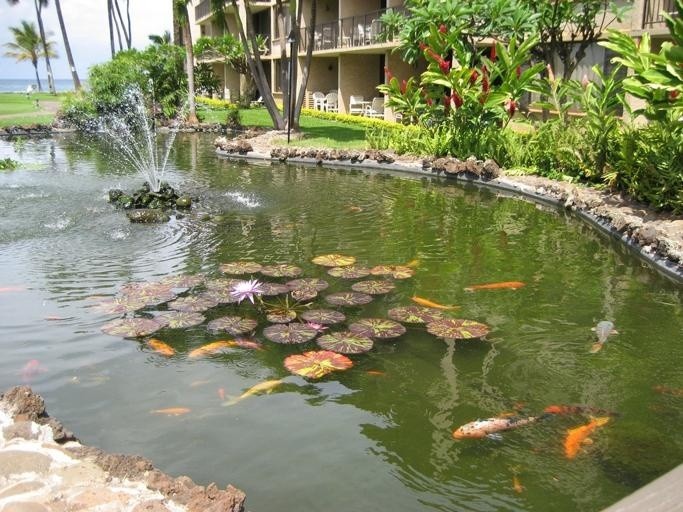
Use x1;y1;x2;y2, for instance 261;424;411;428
369;19;382;44
395;112;402;124
322;94;338;114
349;94;364;115
358;24;369;46
313;92;325;110
321;27;336;49
364;97;384;120
353;27;358;46
341;28;352;48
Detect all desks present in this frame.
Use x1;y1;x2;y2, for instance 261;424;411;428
315;98;327;111
251;101;264;108
361;102;372;119
